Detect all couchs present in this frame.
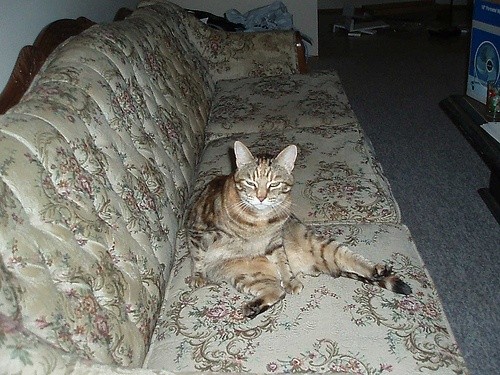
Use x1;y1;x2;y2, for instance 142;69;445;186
0;0;469;375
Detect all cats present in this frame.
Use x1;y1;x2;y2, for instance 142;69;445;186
182;140;413;319
